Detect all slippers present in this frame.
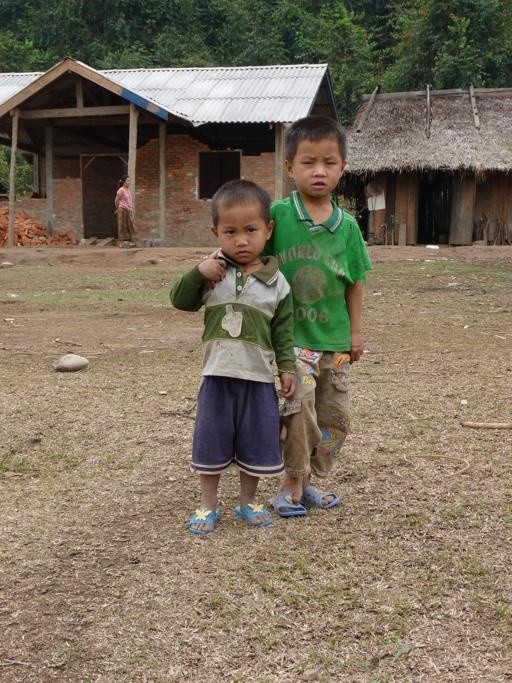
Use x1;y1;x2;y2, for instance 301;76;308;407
234;503;272;526
304;484;342;510
272;491;306;519
187;504;221;535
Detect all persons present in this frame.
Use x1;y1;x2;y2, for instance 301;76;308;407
264;115;375;518
113;173;139;247
168;177;296;536
